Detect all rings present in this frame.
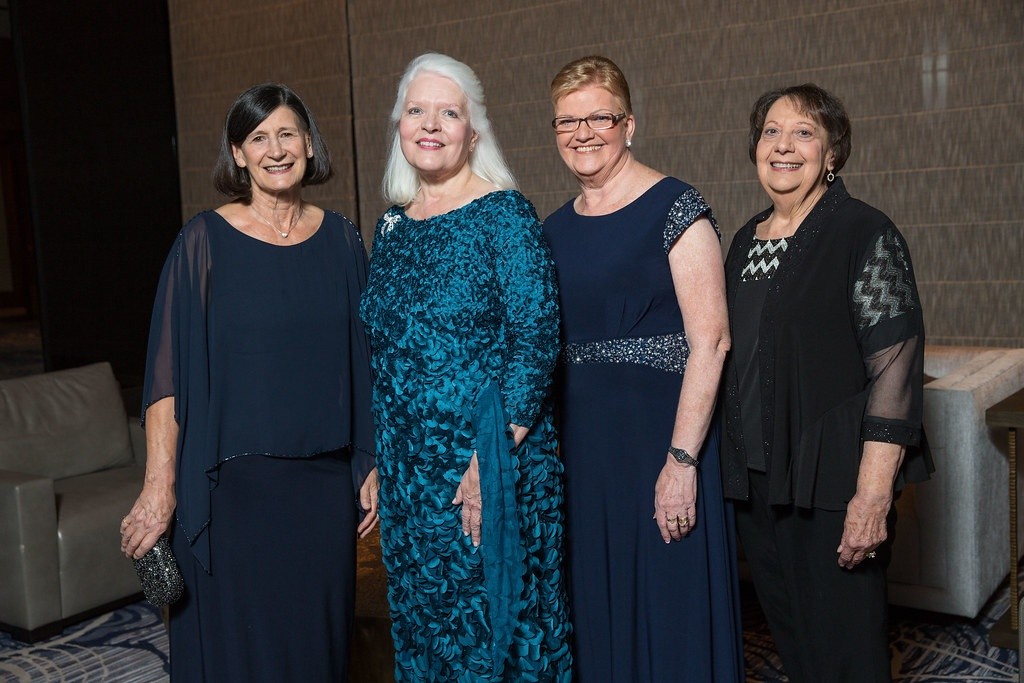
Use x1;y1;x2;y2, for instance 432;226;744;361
123;519;131;526
867;553;876;558
668;519;677;524
679;518;688;527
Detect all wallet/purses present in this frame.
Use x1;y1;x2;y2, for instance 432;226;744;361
131;534;185;609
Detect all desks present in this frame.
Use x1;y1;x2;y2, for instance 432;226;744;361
986;387;1024;650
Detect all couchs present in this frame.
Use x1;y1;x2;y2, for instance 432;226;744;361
0;363;146;645
736;345;1024;628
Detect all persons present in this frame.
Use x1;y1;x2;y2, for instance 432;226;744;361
359;53;574;683
541;56;743;682
117;85;378;683
718;84;938;682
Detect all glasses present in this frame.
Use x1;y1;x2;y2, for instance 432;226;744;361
551;112;625;134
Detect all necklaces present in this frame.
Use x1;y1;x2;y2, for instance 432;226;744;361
254;205;304;237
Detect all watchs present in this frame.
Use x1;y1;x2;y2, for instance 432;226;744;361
669;447;700;467
